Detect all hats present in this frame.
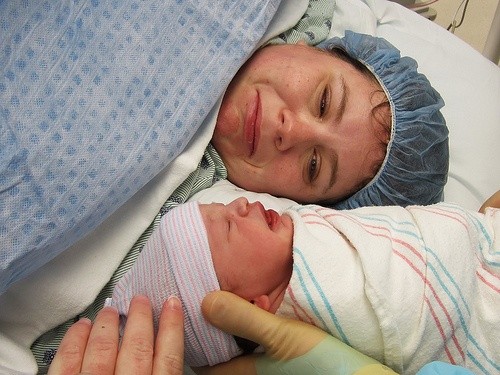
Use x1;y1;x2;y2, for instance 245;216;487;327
101;201;244;367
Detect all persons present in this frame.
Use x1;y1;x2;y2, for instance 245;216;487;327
109;197;500;375
0;0;450;375
